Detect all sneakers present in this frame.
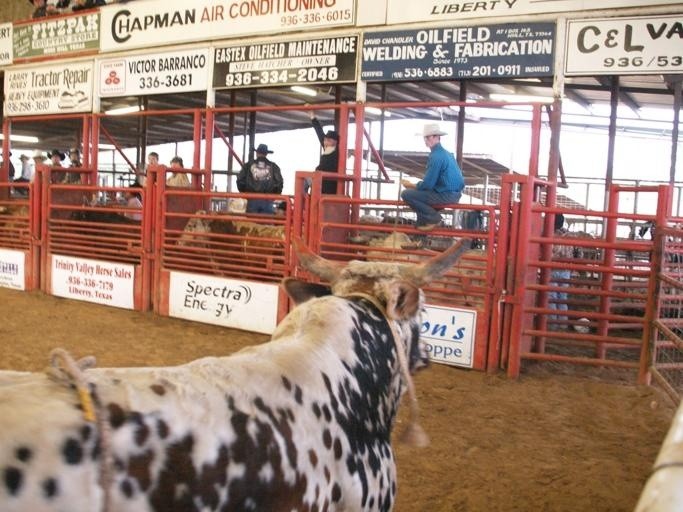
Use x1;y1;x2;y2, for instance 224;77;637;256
416;216;443;231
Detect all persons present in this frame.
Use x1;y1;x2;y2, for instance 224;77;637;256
303;103;339;194
62;148;82;184
0;150;15;182
236;143;283;214
46;148;65;184
12;154;31;196
135;152;158;181
165;156;190;187
29;150;45;185
108;192;120;216
399;123;464;231
122;191;142;221
546;213;582;331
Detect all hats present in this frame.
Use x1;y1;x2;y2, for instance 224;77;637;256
251;143;274;155
320;129;340;141
412;122;448;138
0;146;81;162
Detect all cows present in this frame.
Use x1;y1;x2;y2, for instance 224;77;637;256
345;231;496;312
0;232;474;512
176;210;294;282
59;194;142;265
